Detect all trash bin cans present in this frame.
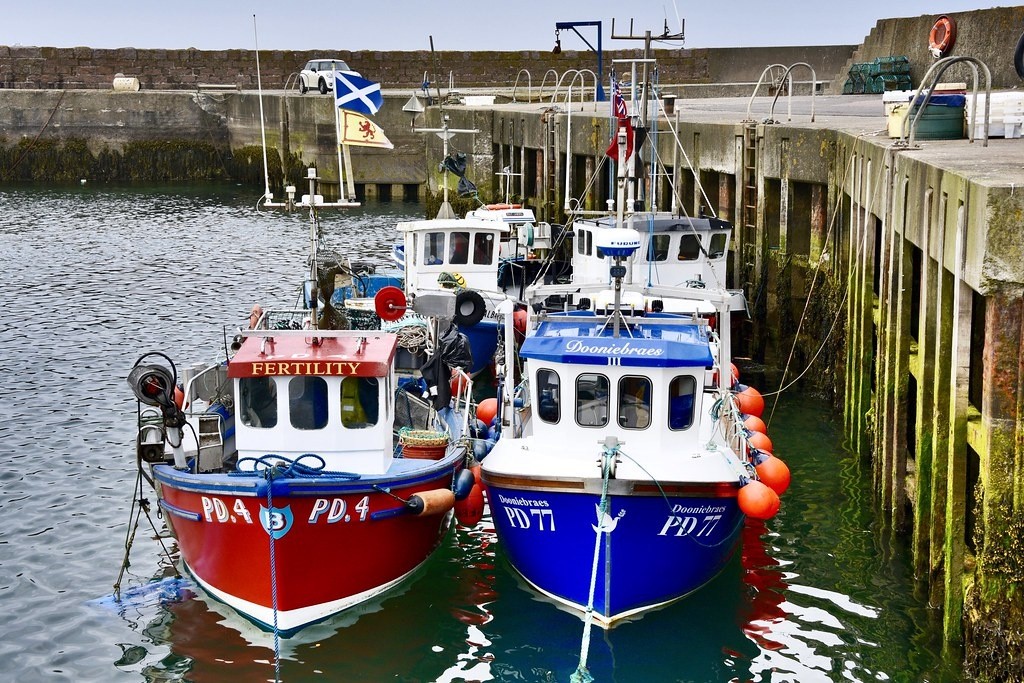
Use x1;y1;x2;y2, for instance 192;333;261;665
908;95;964;141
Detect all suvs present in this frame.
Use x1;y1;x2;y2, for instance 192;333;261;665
298;58;362;94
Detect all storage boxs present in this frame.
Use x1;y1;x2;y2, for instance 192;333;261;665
344;296;376;312
594;289;645;318
882;82;1024;140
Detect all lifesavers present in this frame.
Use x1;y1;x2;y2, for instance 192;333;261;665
249;304;266;330
487;204;522;210
455;290;487;327
928;18;951;51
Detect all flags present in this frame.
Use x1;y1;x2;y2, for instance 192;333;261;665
333;70;384;114
611;73;627;120
336;107;396;149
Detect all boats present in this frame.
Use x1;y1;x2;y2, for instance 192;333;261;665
123;11;476;640
332;13;754;625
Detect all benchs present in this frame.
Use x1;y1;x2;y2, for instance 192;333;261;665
197;81;242;90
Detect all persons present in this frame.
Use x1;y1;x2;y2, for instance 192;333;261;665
427;255;443;265
